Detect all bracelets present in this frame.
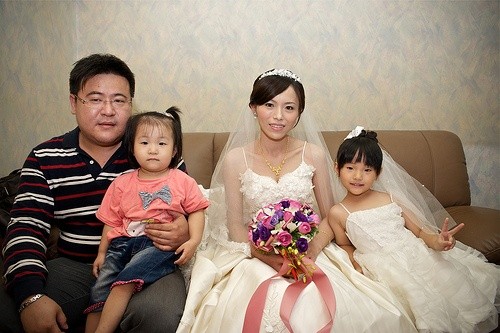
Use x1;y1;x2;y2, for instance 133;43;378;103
18;293;44;313
418;224;425;238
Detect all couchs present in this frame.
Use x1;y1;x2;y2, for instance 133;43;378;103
182;129;500;267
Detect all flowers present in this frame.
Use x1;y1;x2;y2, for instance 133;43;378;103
248;200;320;283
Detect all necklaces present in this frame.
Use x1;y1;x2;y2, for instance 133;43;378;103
258;135;289;175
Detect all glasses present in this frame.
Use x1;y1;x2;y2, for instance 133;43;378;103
74;93;134;108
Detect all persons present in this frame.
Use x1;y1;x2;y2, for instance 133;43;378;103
0;54;187;333
221;69;398;333
84;110;209;333
328;131;500;333
